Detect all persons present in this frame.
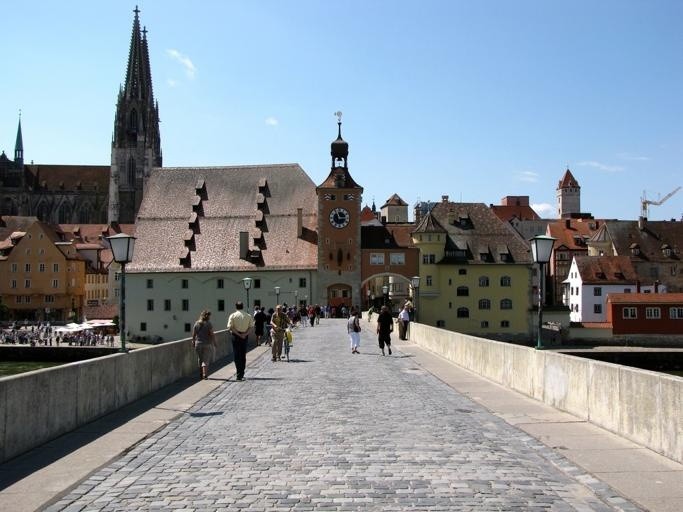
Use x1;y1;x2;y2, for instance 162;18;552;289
376;304;395;356
226;300;254;382
0;317;115;348
346;310;361;355
397;303;411;341
269;304;294;361
251;298;375;348
191;310;218;380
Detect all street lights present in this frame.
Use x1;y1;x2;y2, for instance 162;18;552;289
241;277;253;314
294;289;298;307
371;294;375;312
43;306;53;345
105;232;136;353
411;276;421;322
367;289;371;310
273;286;280;306
529;234;559;350
382;285;389;305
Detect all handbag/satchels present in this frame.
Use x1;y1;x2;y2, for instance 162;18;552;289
353;323;361;332
285;329;292;343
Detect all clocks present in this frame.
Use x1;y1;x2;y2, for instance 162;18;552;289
328;207;350;229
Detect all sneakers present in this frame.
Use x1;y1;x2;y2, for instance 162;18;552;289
200;374;208;380
236;376;245;382
352;349;360;354
381;348;392;356
271;356;281;362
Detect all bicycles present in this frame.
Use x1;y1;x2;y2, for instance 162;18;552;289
281;329;292;362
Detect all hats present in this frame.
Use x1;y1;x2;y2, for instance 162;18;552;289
276;305;282;310
